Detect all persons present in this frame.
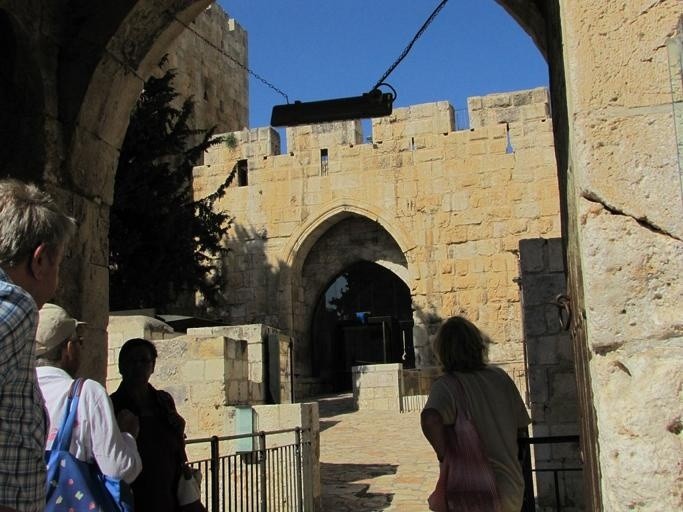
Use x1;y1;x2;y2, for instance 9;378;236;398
33;299;141;511
102;335;207;511
0;176;73;510
420;314;537;511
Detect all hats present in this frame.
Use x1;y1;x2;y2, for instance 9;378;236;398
35;303;88;357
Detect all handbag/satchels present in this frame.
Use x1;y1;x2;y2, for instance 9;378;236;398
42;375;136;511
428;372;504;511
176;464;207;512
